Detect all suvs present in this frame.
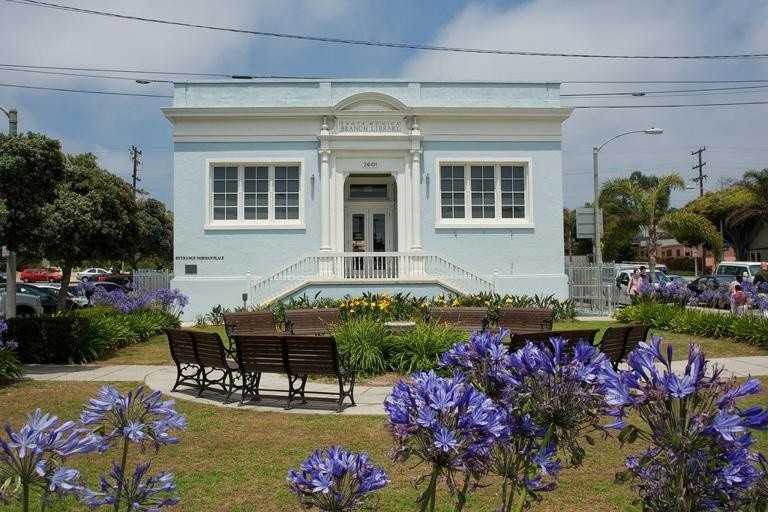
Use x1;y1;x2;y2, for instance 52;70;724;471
713;260;767;284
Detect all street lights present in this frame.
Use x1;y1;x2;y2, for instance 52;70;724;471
685;185;723;253
136;77;174;85
588;127;664;266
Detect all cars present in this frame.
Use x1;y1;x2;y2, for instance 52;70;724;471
603;260;688;305
686;273;738;309
75;267;111;282
20;269;63;283
0;273;133;317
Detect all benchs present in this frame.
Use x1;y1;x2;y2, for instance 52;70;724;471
219;310;293;363
425;305;490;337
483;307;554;337
501;328;601;379
591;322;654;374
279;308;343;336
228;331;357;414
159;326;256;404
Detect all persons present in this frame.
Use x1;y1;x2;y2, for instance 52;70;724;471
732;285;745;314
638;266;653;295
729;275;743;314
753;261;768;306
627;268;642;300
